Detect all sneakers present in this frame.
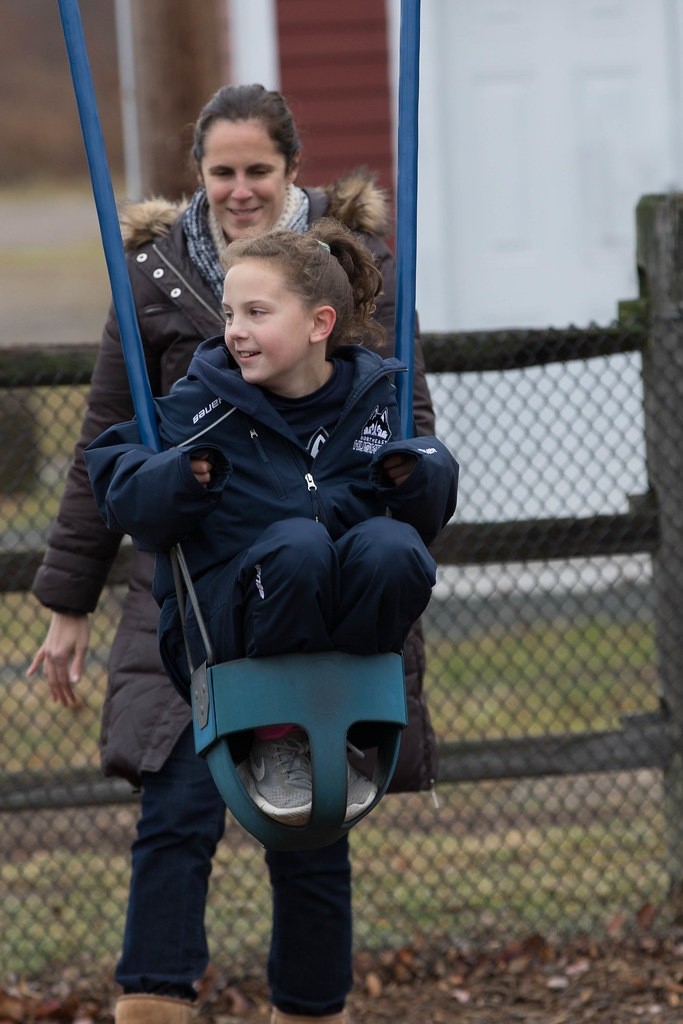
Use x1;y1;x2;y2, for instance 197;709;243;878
344;762;379;819
245;732;317;817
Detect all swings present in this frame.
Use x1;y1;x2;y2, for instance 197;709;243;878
54;0;425;854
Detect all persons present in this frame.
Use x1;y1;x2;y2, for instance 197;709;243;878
81;213;459;828
28;80;435;1024
